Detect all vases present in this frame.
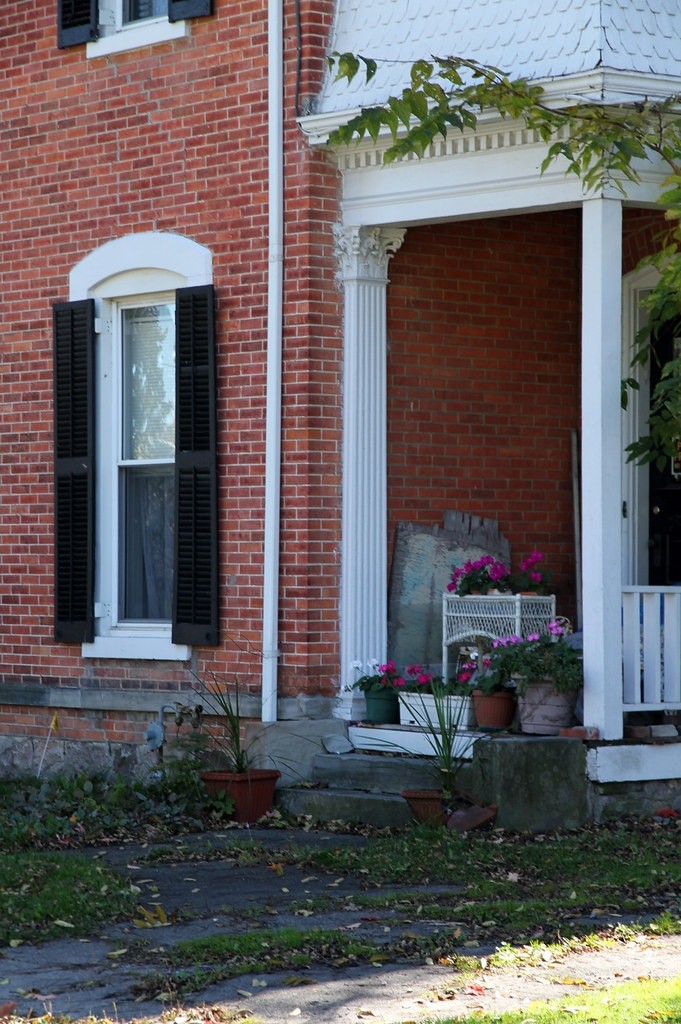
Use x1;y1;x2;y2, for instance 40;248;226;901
509;671;583;738
518;589;539;598
466;585;484;597
475;684;518;734
489;586;513;597
397;689;477;732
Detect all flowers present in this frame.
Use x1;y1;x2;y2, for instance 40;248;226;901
486;620;585;695
377;660;493;698
486;559;514;591
516;550;544;593
471;645;517;688
446;553;493;595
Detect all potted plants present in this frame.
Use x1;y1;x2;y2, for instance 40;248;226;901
344;665;399;721
172;660;326;828
354;659;517;833
